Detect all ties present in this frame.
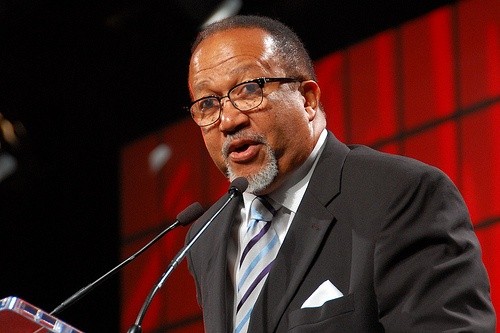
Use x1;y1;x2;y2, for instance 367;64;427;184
234;195;284;333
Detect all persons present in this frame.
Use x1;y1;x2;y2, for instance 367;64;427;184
184;16;499;333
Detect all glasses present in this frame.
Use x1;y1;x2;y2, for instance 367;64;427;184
185;77;298;127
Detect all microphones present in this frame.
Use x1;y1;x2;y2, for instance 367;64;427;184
51;202;203;317
126;177;249;333
